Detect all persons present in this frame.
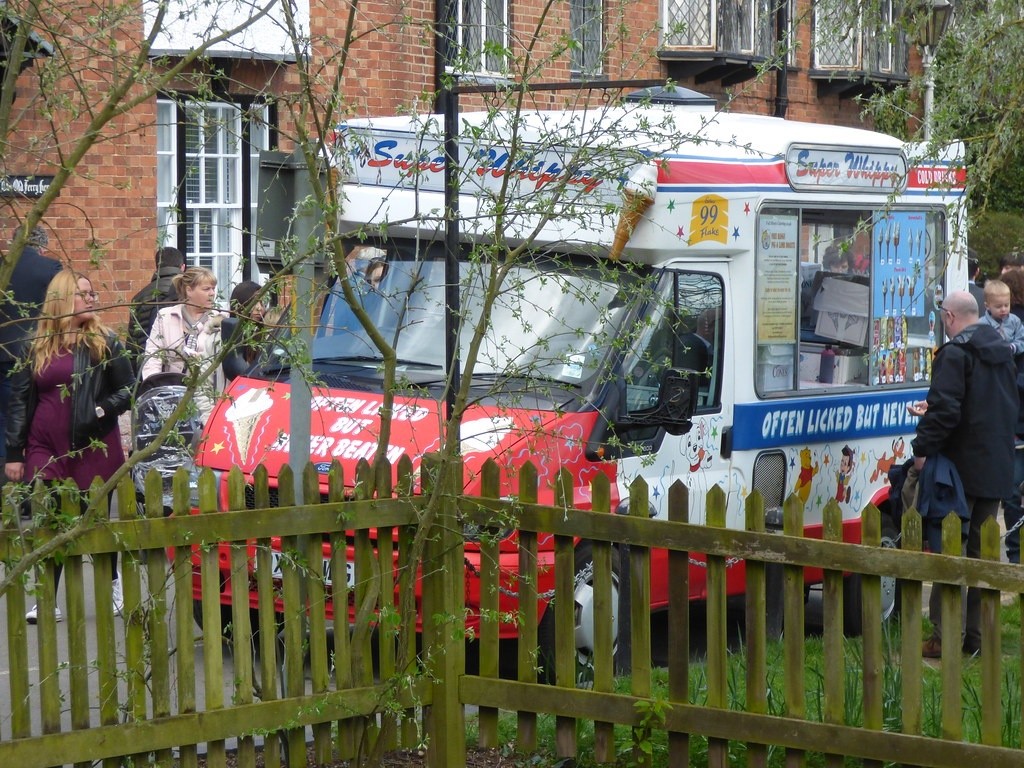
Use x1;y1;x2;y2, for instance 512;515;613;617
967;248;1024;600
823;246;856;274
908;288;1021;658
0;225;280;620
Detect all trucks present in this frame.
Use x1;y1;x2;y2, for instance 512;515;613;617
185;101;968;683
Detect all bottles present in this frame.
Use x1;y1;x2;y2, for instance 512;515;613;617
819;344;834;383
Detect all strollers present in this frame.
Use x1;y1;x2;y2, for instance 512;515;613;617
130;370;205;560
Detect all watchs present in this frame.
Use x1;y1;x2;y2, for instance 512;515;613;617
95;405;105;418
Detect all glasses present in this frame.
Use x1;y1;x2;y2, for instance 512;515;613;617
75;291;100;302
937;301;955;318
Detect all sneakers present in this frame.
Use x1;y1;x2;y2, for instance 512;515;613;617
922;636;942;657
963;646;981;655
26;605;62;624
111;571;124;615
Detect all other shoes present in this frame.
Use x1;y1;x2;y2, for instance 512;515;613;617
1015;435;1024;449
